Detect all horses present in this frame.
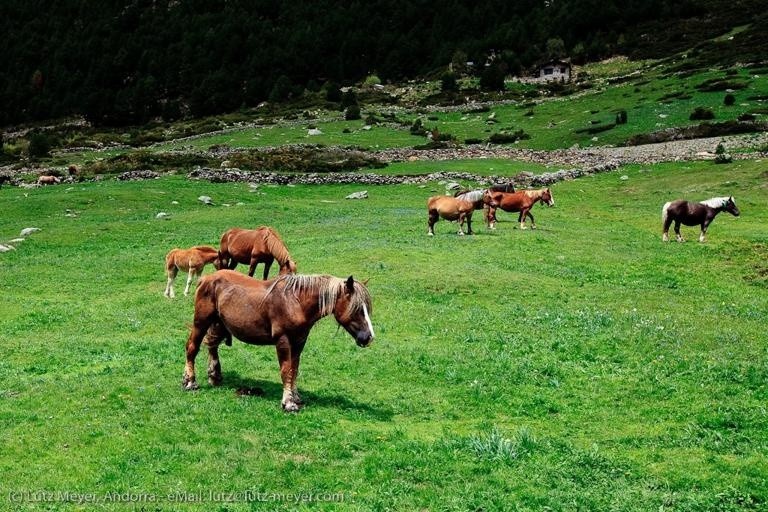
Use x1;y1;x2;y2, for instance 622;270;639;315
182;270;375;411
164;245;227;299
454;183;515;223
221;225;297;281
487;187;554;230
661;194;740;243
426;189;497;236
